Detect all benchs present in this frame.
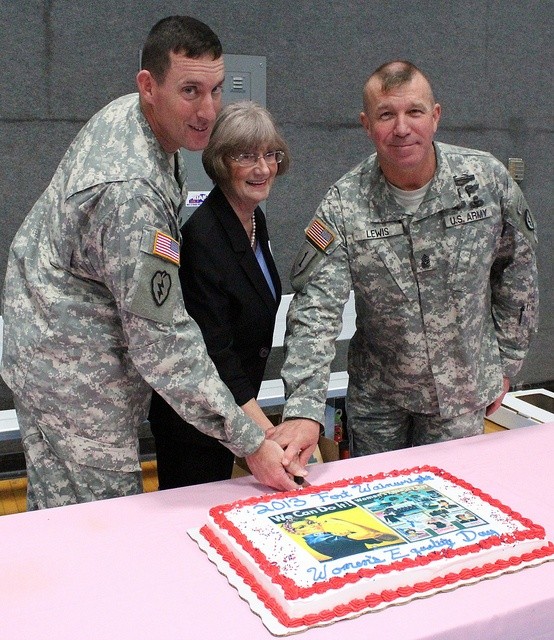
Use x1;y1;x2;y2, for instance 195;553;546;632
0;290;357;441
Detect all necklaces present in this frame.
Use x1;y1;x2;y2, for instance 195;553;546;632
250;212;256;248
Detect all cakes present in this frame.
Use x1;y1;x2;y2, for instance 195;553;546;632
198;466;554;626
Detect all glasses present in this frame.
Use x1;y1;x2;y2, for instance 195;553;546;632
225;150;286;167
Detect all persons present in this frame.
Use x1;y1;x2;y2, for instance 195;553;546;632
282;518;383;558
437;500;458;510
405;529;430;539
455;514;478;523
147;99;290;490
265;60;540;467
0;16;308;513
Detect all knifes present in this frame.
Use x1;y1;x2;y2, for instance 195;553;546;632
293;475;313;494
